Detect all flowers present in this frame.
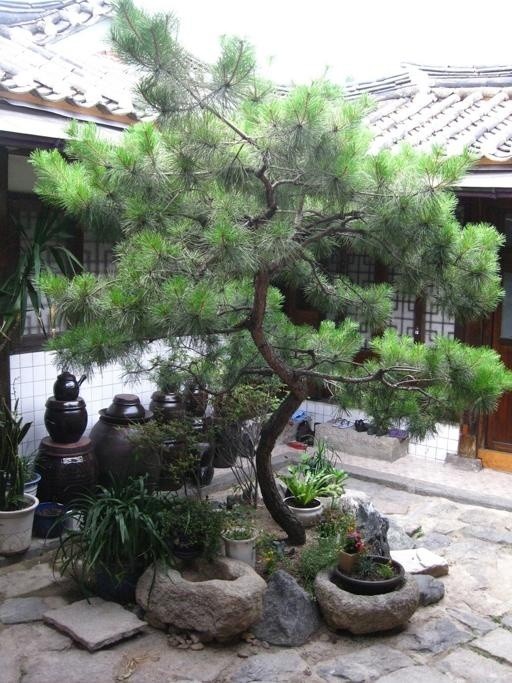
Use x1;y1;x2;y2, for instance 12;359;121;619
344;531;364;547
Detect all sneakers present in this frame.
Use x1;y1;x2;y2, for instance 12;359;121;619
355;419;407;437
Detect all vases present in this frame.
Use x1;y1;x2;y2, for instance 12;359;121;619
336;548;359;574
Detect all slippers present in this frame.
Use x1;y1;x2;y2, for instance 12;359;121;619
333;417;352;428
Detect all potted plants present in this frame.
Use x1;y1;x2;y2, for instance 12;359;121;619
276;433;348;527
0;397;66;560
78;478;263;606
332;553;405;596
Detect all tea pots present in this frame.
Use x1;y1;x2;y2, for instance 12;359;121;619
52;370;88;399
